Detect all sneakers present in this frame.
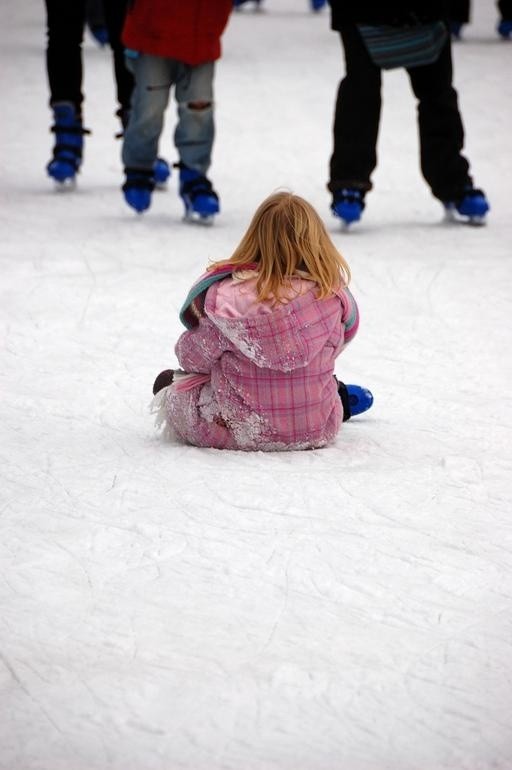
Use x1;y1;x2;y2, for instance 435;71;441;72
329;186;366;221
342;384;374;424
440;183;489;214
123;167;154;212
173;161;221;215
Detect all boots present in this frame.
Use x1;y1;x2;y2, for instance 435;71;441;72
112;105;171;181
45;95;92;181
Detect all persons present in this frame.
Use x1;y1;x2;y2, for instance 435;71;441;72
154;192;373;452
325;0;489;217
450;0;511;38
122;0;238;216
41;1;170;182
